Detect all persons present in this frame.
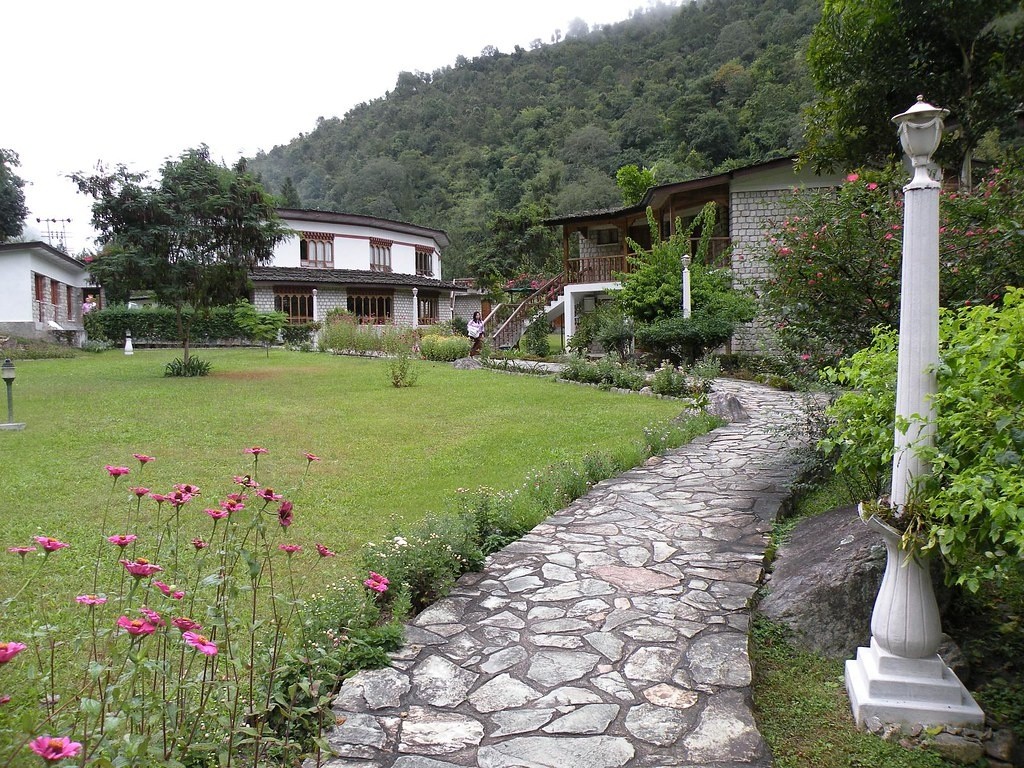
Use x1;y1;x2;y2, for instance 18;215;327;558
81;298;92;330
467;312;485;358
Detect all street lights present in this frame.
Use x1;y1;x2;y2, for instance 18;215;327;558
312;289;318;322
411;288;419;330
845;94;985;729
681;254;693;319
